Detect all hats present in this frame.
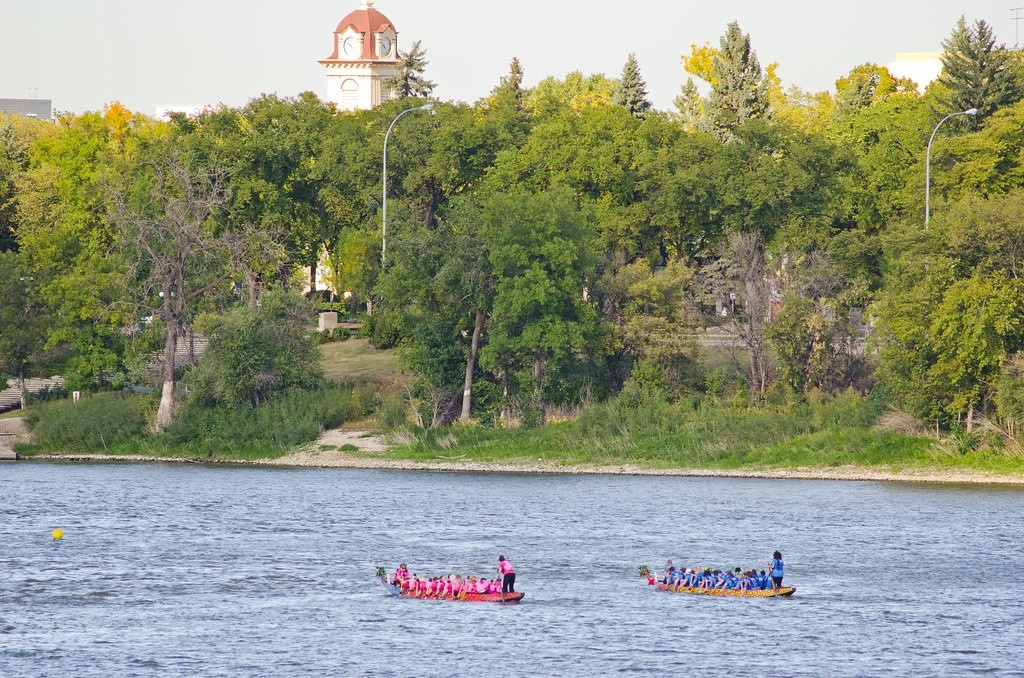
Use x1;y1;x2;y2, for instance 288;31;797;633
499;555;505;560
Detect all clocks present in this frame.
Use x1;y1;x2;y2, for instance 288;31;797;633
344;38;358;57
380;37;392;57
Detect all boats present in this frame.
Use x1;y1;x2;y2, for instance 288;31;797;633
375;568;525;605
640;566;798;600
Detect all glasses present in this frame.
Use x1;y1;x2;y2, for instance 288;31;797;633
401;567;406;569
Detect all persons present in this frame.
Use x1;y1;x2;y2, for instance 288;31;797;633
663;560;770;595
394;563;504;599
498;555;515;593
767;551;783;587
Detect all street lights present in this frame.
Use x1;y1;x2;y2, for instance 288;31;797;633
378;104;439;270
926;108;978;233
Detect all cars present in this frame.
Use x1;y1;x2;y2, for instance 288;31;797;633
304;287;342;302
